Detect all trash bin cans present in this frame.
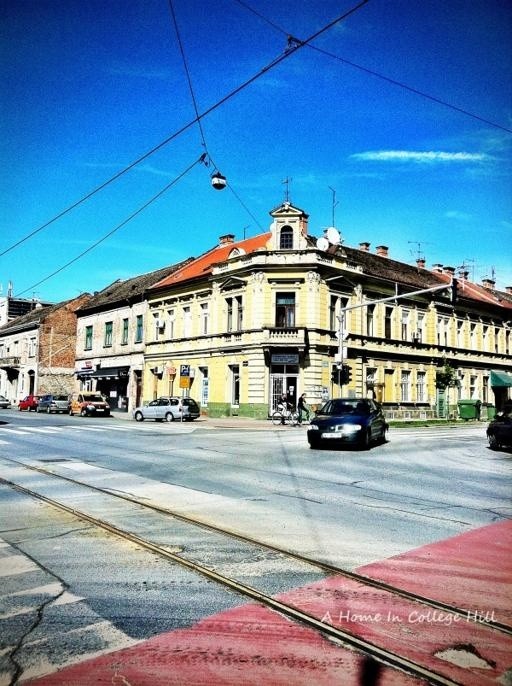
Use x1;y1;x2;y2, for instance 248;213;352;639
457;399;496;422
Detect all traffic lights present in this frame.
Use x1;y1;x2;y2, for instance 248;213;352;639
343;364;352;384
331;365;339;384
451;278;460;304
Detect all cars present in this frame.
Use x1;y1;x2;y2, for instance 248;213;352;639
0;391;110;419
485;398;511;451
305;398;388;450
133;396;200;421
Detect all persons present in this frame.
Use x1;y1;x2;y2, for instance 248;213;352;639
276;393;294;425
297;392;309;424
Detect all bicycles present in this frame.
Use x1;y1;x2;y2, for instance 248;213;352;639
271;405;299;426
291;405;317;425
